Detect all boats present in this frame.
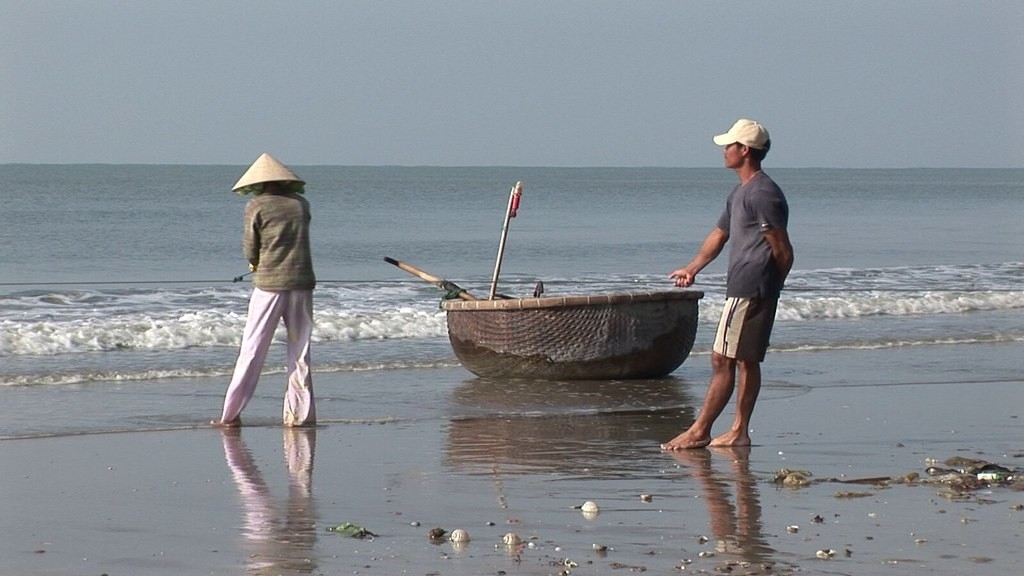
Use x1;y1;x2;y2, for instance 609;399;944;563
438;179;704;382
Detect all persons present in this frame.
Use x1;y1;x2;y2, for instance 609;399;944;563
217;429;317;576
658;120;794;451
663;449;762;561
211;154;316;427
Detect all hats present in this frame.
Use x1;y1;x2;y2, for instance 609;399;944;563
232;152;307;192
713;119;768;149
